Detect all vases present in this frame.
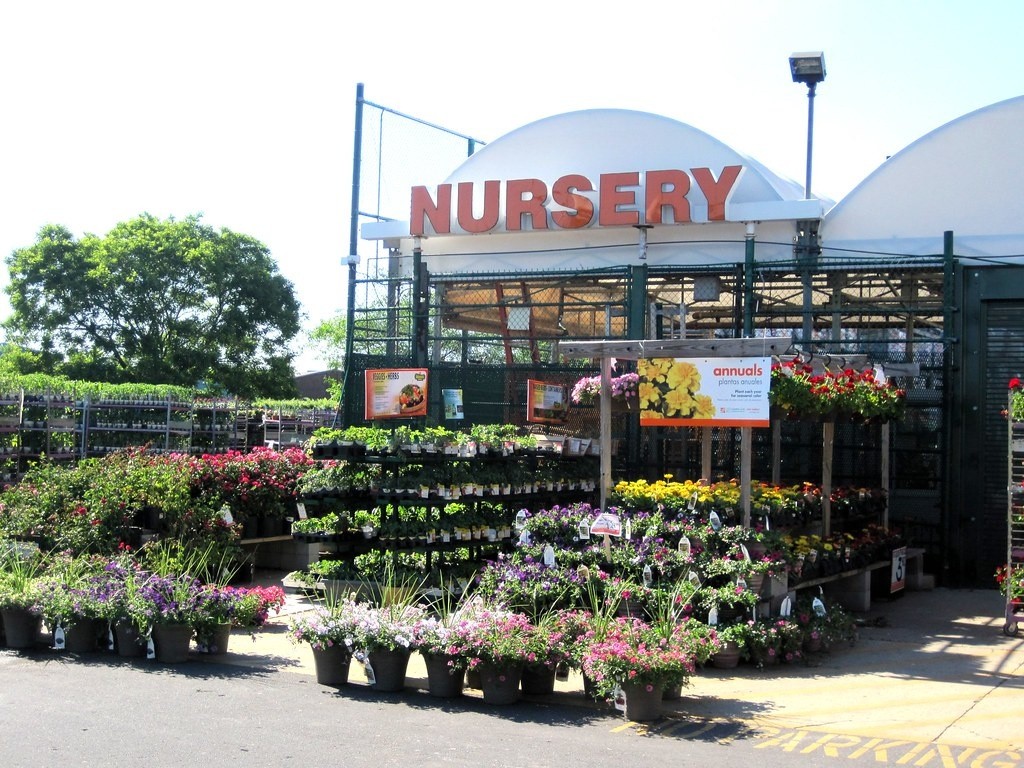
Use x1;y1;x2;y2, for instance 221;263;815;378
786;406;800;420
581;665;611;702
814;408;831;422
367;644;410;691
740;571;765;595
194;621;231;654
151;623;192;662
421;650;468;696
111;618;149;656
594;397;615;413
780;638;802;663
663;674;682;700
750;642;776;665
62;612;96;652
480;660;521;706
801;645;823;667
622;670;663;720
92;617;109;650
614;684;628;711
522;660;556;704
467;665;482;689
310;639;351;684
249;613;265;626
610;399;632;416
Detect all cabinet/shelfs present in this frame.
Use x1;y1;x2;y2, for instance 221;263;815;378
0;391;340;493
281;433;622;610
1003;387;1024;636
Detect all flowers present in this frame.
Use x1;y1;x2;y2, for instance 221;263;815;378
768;356;906;420
800;612;828;648
772;617;802;660
639;358;716;419
572;372;638;404
746;620;781;645
581;615;721;699
720;555;774;574
34;563;284;631
290;589;594;660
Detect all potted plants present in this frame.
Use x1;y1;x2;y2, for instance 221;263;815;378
289;423;602;591
712;624;745;669
0;372;339;472
1;541;48;648
